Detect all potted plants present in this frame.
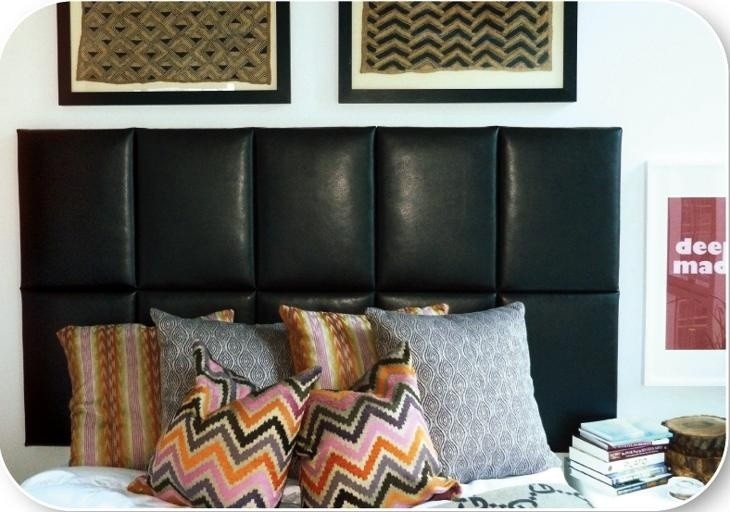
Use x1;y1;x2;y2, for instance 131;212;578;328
645;162;729;388
339;1;577;103
56;2;291;106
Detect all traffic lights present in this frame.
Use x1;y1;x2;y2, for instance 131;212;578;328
278;303;450;392
294;339;462;508
149;307;291;430
118;334;323;509
56;309;235;469
363;301;564;485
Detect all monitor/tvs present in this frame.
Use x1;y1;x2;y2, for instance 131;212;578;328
569;415;674;498
